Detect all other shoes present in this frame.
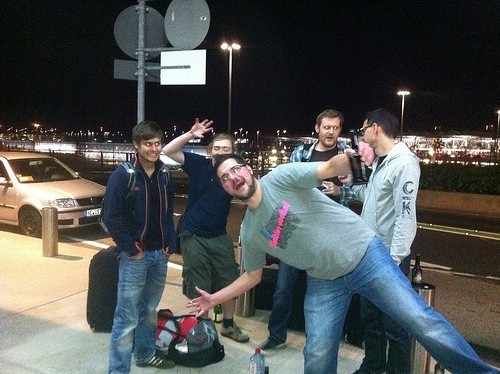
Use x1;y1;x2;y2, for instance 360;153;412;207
259;336;287;354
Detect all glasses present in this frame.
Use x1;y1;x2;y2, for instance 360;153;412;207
357;124;372;136
220;164;245;184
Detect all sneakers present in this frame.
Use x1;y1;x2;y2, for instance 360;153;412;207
135;353;176;369
221;322;250;342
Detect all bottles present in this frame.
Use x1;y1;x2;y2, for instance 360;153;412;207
249;348;265;374
213;304;223;323
412;254;422;283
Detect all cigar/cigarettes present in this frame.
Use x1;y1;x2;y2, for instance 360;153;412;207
323;184;329;189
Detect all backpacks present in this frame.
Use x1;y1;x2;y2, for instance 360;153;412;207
156;309;225;368
97;162;137;237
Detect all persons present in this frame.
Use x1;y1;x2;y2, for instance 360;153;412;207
188;148;500;374
163;118;249;343
258;109;354;352
320;109;421;374
102;120;176;374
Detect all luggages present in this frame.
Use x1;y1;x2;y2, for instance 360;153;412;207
86;246;120;333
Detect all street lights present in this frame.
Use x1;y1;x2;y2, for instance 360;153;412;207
397;91;411;133
220;42;241;135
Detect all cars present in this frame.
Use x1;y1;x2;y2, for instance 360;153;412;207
0;151;107;239
